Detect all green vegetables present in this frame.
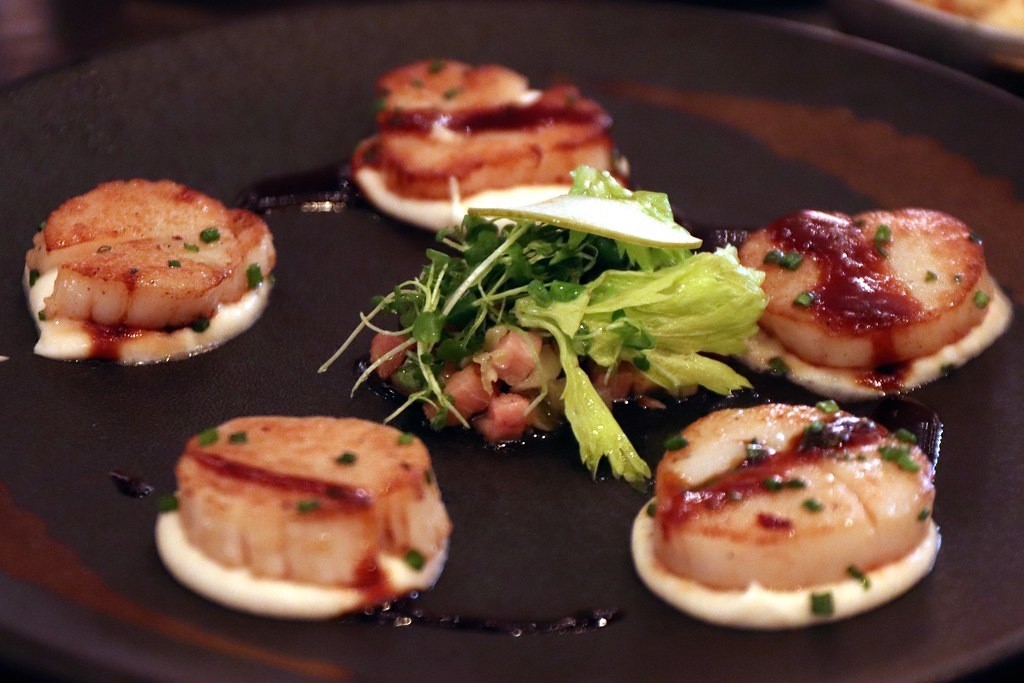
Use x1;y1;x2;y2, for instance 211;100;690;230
313;166;771;490
